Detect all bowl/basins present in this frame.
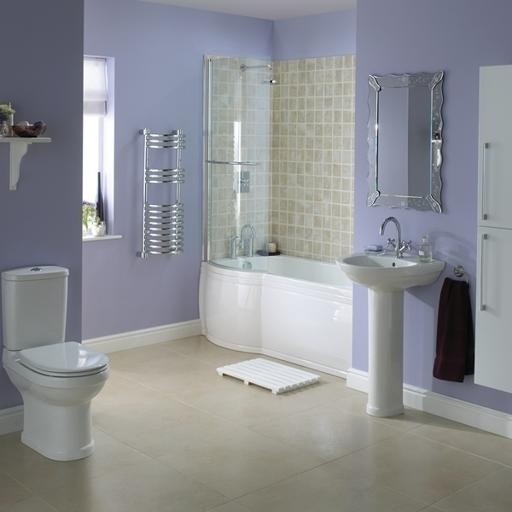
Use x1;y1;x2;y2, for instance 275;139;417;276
10;124;47;138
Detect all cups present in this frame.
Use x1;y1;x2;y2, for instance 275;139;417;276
0;110;16;137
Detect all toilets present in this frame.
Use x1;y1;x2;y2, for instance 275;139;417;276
1;266;111;462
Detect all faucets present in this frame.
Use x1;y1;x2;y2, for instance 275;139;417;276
230;235;240;258
241;224;256;249
379;216;400;247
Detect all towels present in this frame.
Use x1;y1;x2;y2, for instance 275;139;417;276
432;277;474;382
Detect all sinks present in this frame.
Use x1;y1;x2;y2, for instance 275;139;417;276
335;253;445;289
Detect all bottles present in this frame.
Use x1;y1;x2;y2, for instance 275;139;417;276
418;235;433;263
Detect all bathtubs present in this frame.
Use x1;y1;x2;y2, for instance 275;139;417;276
198;254;354;380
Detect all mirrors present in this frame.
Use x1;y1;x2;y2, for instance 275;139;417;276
367;71;445;212
